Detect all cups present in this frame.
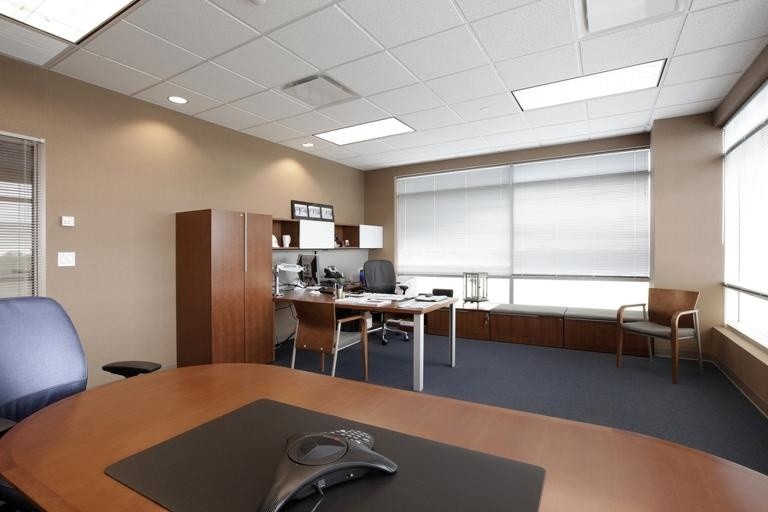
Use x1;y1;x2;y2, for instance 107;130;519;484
336;284;344;299
360;270;364;284
282;234;291;247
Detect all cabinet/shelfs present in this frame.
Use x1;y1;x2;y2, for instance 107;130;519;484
177;209;274;367
272;217;384;251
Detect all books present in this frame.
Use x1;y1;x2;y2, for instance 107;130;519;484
336;290;448;314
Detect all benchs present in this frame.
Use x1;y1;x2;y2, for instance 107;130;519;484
426;296;650;356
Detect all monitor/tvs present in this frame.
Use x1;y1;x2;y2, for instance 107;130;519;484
298;255;317;284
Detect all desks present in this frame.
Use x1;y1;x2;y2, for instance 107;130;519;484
272;285;456;393
0;362;766;512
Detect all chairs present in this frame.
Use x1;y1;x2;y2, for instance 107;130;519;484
614;287;704;384
0;297;162;436
362;258;410;348
287;297;370;382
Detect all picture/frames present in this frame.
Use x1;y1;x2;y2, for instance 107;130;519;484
290;200;308;219
305;202;322;220
320;204;333;221
